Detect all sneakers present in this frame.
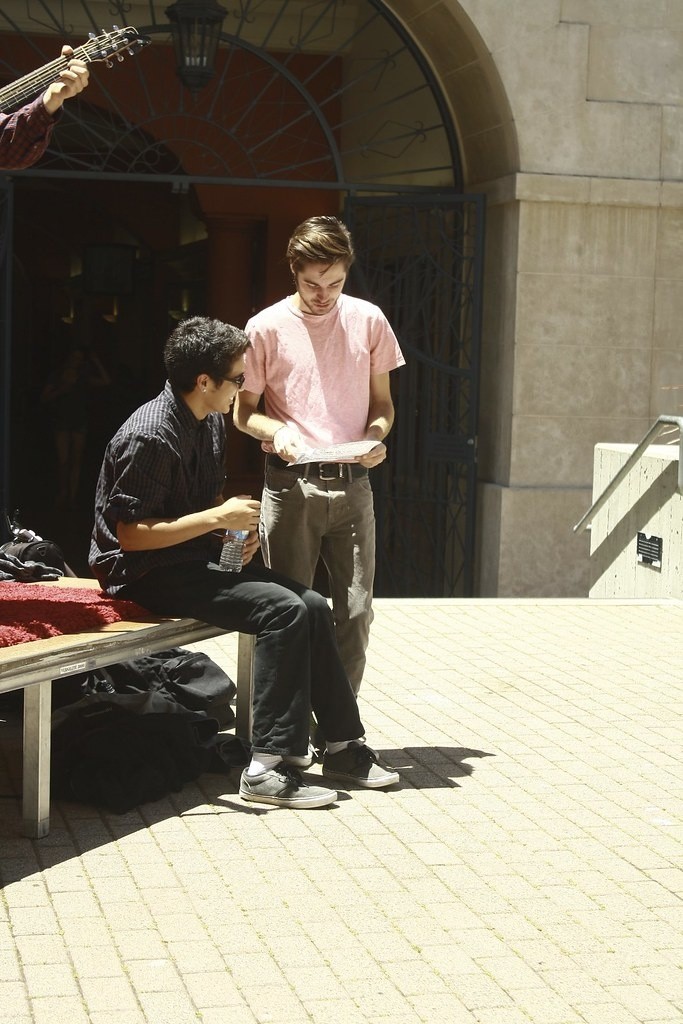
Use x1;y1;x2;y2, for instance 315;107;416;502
322;736;400;788
238;760;338;808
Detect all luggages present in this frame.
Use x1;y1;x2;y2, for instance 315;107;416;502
0;539;67;577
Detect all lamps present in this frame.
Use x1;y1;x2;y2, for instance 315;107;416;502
163;1;229;107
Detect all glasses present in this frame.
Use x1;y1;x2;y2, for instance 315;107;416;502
224;372;246;390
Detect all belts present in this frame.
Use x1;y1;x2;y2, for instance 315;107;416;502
266;454;368;480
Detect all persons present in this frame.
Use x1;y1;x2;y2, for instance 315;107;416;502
0;45;90;171
89;315;400;808
41;345;112;515
231;216;407;766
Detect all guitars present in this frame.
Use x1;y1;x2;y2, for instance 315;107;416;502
0;24;153;115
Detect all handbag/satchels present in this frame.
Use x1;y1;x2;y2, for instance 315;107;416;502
52;645;237;727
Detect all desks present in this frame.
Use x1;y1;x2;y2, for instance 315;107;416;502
0;576;257;840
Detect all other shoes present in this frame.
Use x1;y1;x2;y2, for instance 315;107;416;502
282;735;320;767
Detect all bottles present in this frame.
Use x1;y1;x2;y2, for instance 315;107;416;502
219;529;249;573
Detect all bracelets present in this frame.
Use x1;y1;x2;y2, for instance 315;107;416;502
272;424;287;444
